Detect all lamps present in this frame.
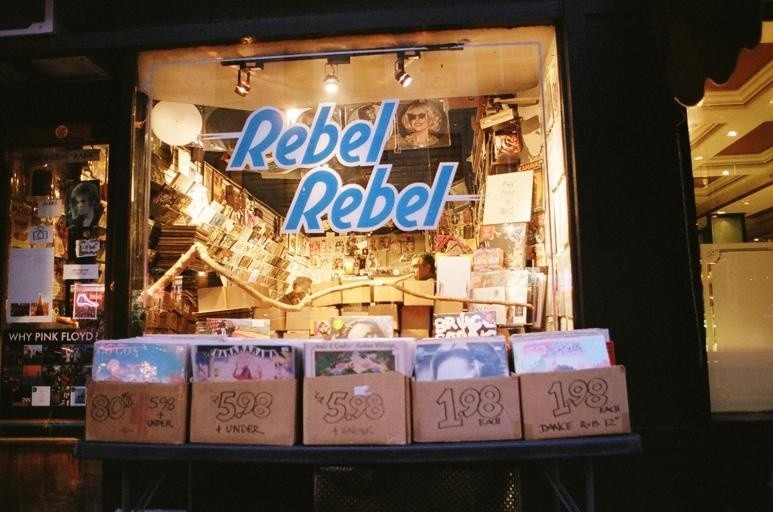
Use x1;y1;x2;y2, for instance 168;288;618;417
233;52;421;97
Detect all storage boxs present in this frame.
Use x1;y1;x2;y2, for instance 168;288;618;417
301;371;414;447
516;364;631;443
191;377;301;448
411;371;524;445
86;381;191;445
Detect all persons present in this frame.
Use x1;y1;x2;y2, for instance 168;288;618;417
410;252;436;296
429;342;505;382
292;276;312;301
67;182;99;227
345;320;382;339
